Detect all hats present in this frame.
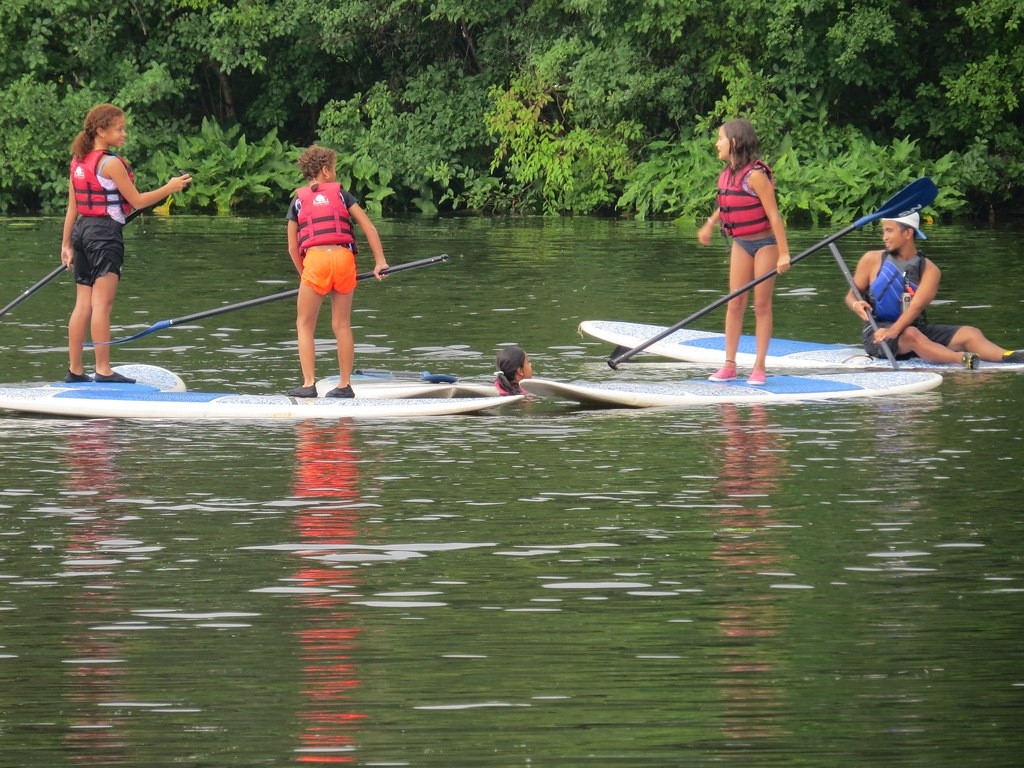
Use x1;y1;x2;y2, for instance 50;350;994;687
882;212;926;240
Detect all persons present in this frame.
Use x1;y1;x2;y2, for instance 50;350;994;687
846;209;1024;370
61;104;192;384
495;348;532;394
698;121;791;384
286;144;389;401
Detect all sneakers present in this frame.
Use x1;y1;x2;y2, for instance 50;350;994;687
962;352;979;370
1002;350;1024;363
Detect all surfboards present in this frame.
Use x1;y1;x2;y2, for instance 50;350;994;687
51;364;187;394
578;316;1024;371
314;370;453;398
520;370;945;412
0;383;521;421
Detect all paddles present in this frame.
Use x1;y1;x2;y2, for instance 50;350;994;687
355;367;458;384
108;254;445;347
608;174;942;368
0;206;148;314
819;233;901;368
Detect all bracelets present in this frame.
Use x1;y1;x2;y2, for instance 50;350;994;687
707;217;717;224
852;301;858;310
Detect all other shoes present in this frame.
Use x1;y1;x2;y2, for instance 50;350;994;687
64;370;93;382
95;372;136;383
325;384;355;398
747;369;766;385
709;368;737;382
285;383;318;397
